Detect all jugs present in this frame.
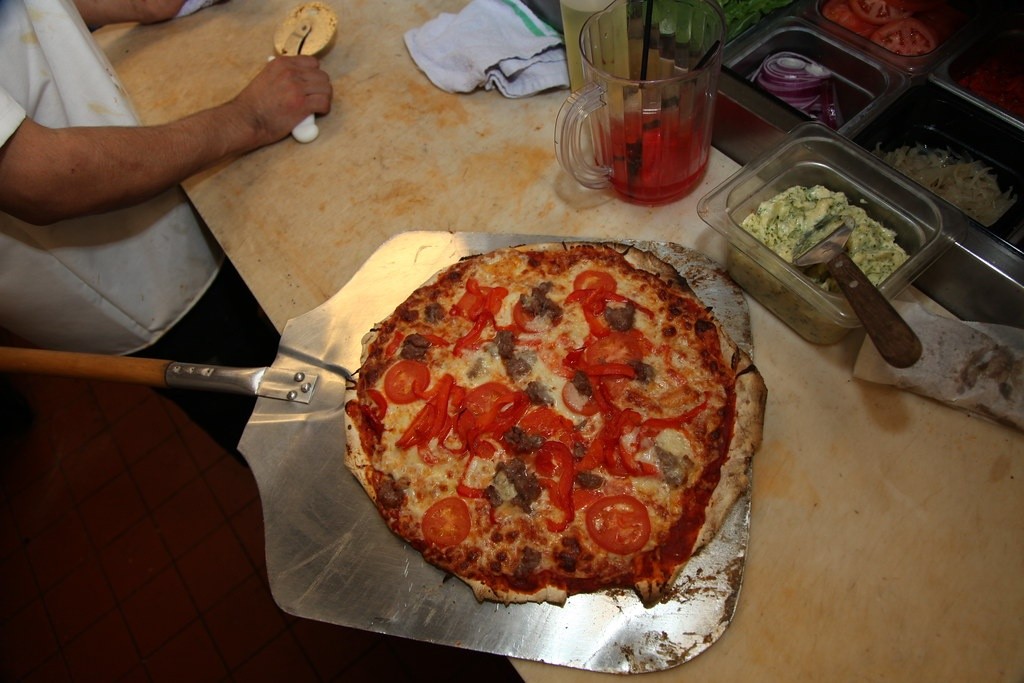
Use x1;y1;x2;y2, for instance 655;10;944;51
555;0;728;205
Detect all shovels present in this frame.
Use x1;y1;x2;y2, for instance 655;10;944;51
0;227;770;675
791;214;927;371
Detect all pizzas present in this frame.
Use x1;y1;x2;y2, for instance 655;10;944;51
342;241;769;608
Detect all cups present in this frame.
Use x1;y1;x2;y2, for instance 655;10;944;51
560;0;630;93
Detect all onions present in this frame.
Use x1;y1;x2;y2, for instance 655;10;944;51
749;52;843;131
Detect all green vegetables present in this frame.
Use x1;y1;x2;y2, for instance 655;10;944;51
627;0;794;54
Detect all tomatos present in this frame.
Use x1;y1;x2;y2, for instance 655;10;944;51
820;0;965;56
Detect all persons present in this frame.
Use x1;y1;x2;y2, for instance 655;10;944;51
0;0;334;463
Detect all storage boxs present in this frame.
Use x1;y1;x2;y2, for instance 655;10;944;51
697;122;969;345
